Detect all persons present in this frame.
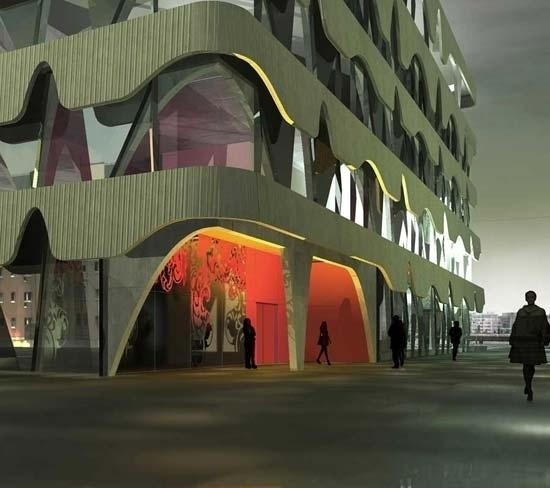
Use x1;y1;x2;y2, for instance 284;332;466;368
316;320;333;364
242;317;258;370
448;320;463;360
388;315;404;368
508;289;549;400
397;318;408;367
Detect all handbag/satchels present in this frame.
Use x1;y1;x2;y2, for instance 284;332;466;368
318;336;329;346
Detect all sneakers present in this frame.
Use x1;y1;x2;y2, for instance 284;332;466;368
525;387;533;400
317;359;331;365
392;360;404;368
245;364;257;369
